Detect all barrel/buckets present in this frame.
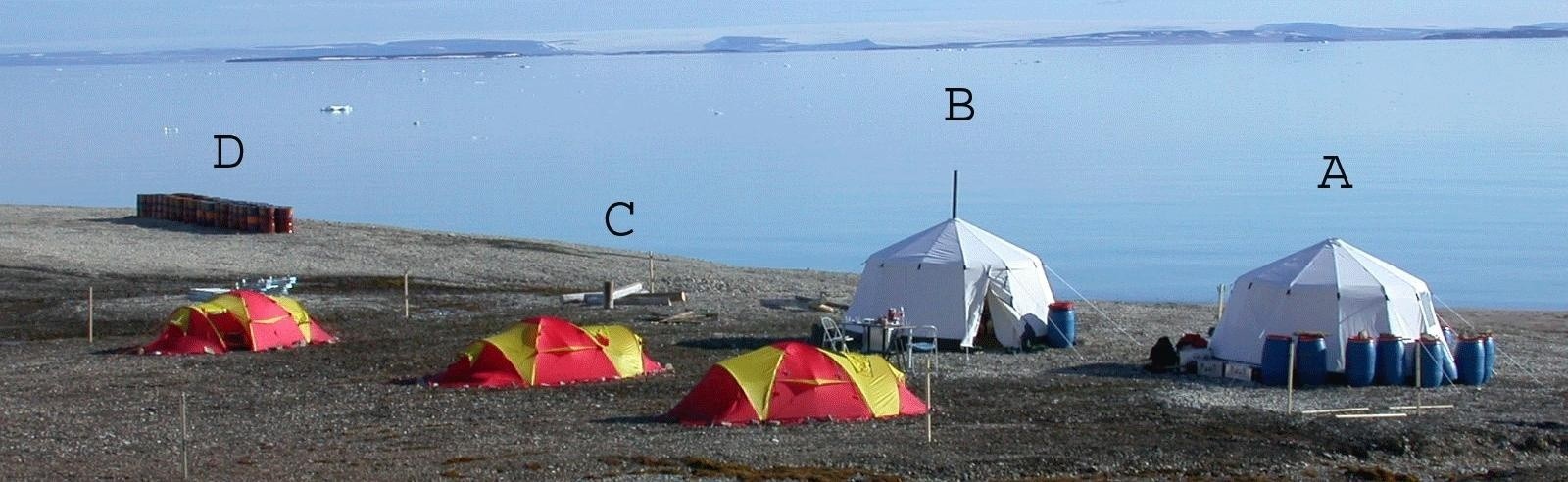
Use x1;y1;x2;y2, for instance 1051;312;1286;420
1047;302;1076;347
1261;326;1497;387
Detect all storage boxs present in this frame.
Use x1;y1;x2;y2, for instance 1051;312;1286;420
1196;357;1262;382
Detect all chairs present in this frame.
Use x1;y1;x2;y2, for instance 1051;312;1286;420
820;317;940;373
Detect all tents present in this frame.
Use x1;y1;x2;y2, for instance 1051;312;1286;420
1208;237;1454;375
841;217;1055;348
145;290;333;357
429;317;665;391
663;341;927;428
